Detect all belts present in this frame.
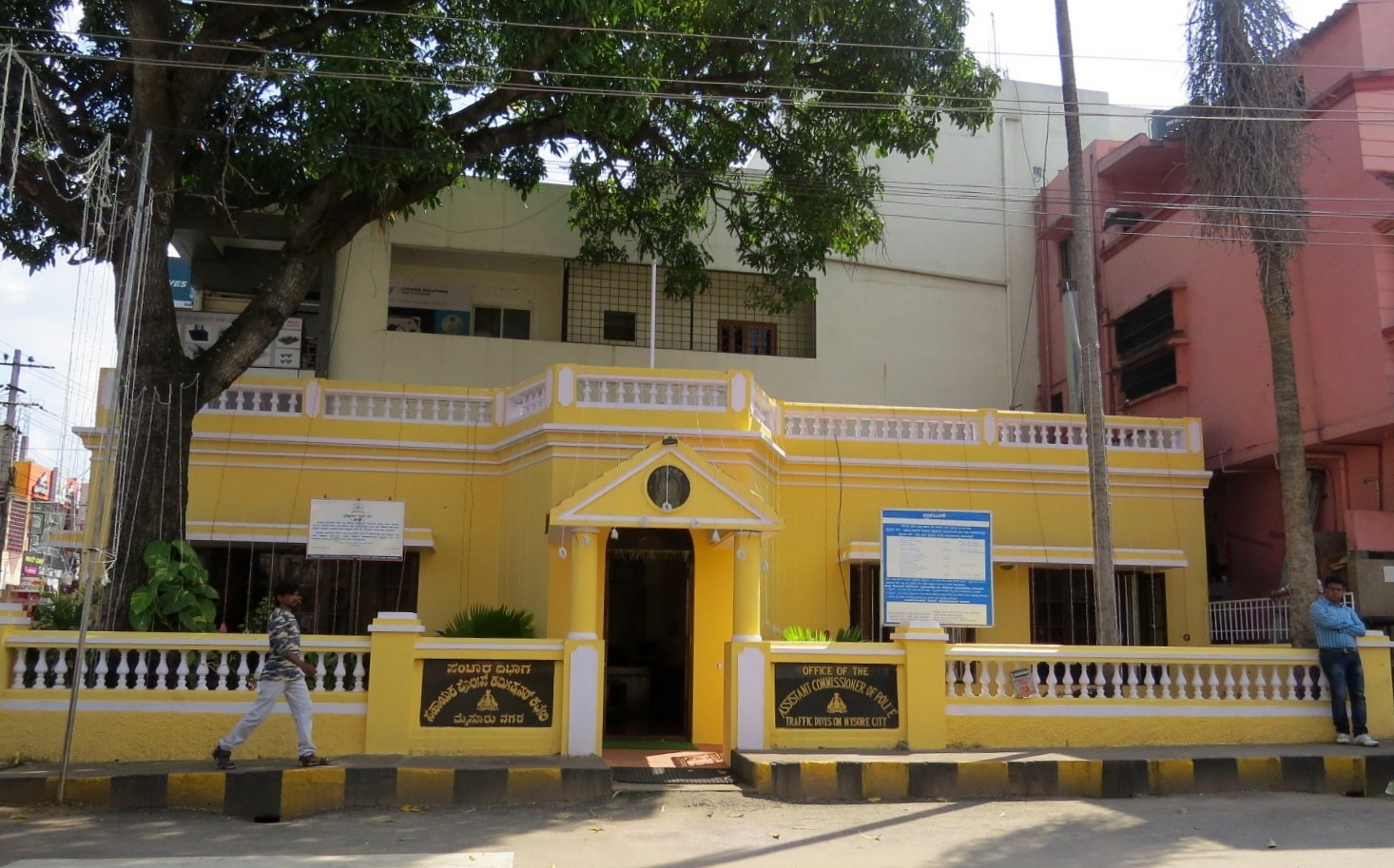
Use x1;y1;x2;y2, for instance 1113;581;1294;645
1320;647;1358;655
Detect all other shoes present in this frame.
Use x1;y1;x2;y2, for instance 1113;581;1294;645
1335;733;1349;745
212;745;236;769
1352;734;1380;748
297;752;331;767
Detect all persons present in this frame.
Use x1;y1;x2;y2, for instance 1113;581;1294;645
1310;576;1379;747
211;582;329;769
